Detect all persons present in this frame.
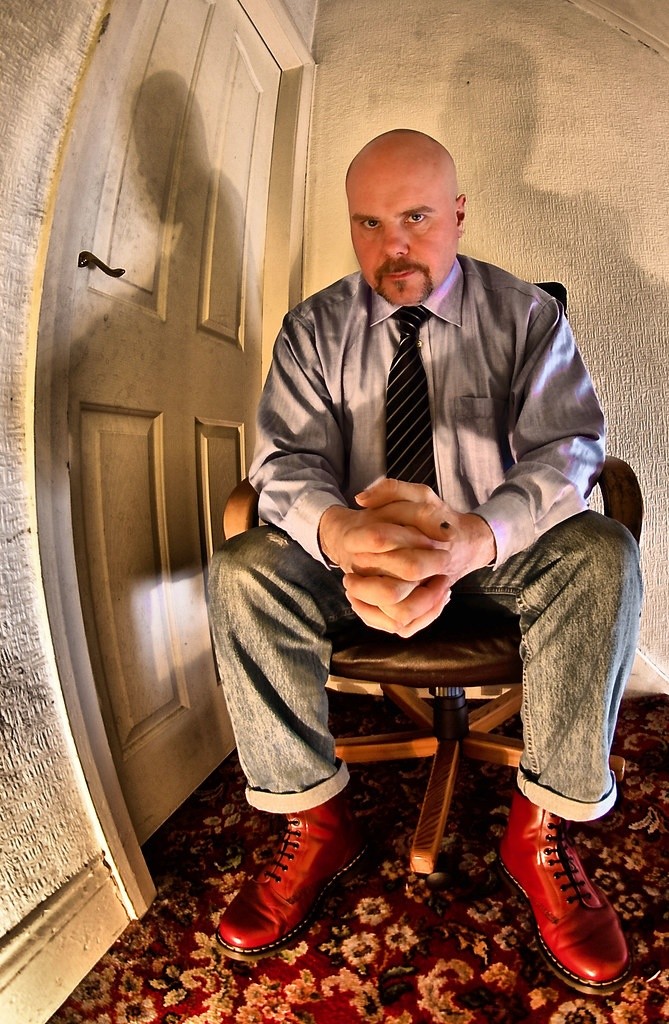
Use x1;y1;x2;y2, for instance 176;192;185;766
198;124;645;998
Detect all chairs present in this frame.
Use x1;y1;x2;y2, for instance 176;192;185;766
222;278;644;890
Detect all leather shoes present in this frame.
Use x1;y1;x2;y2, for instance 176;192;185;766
213;784;375;966
497;787;636;998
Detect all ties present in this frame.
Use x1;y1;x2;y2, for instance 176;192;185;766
383;304;441;500
